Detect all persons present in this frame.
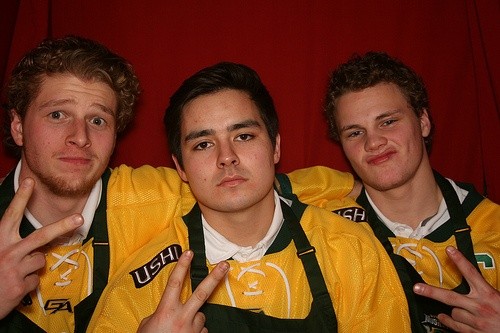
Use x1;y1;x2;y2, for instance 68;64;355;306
1;31;364;333
84;60;413;332
299;49;499;332
